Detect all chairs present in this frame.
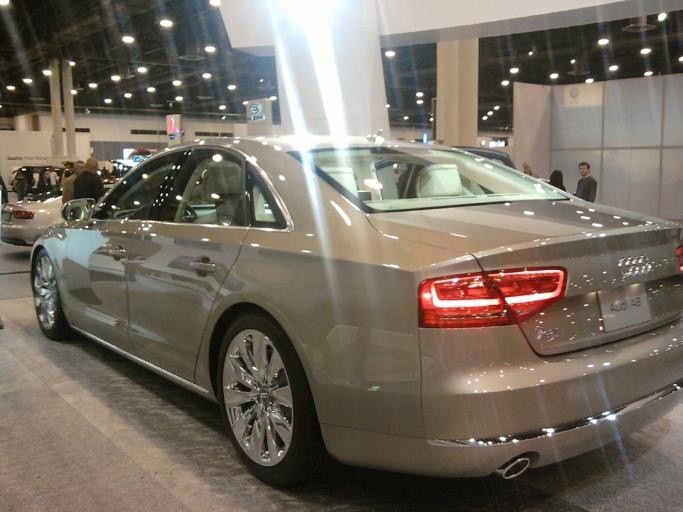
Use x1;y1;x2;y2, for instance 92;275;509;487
319;157;460;225
191;159;245;224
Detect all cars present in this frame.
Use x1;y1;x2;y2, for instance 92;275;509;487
28;135;683;487
0;178;120;246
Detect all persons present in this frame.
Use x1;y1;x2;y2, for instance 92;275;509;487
0;157;132;207
575;162;597;203
522;162;538;178
546;169;566;192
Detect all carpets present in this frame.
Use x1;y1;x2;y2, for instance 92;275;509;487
1;296;683;511
